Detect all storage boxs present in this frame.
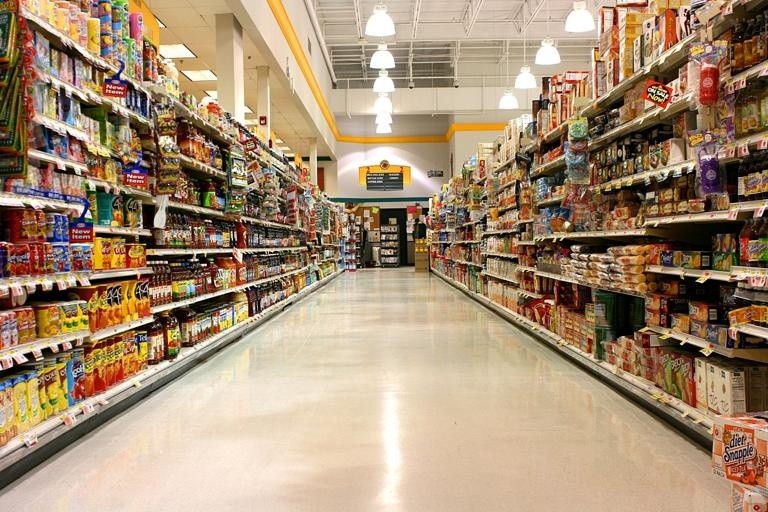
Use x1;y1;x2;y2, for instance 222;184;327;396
415;252;429;273
711;412;768;512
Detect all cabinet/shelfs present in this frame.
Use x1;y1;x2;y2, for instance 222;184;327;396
0;6;343;490
427;0;768;452
343;224;401;270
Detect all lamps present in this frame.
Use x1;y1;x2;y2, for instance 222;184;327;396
364;1;397;135
498;0;596;111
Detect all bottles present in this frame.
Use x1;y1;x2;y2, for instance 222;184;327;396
145;36;181;102
227;113;303;184
147;206;311;365
144;92;228;208
